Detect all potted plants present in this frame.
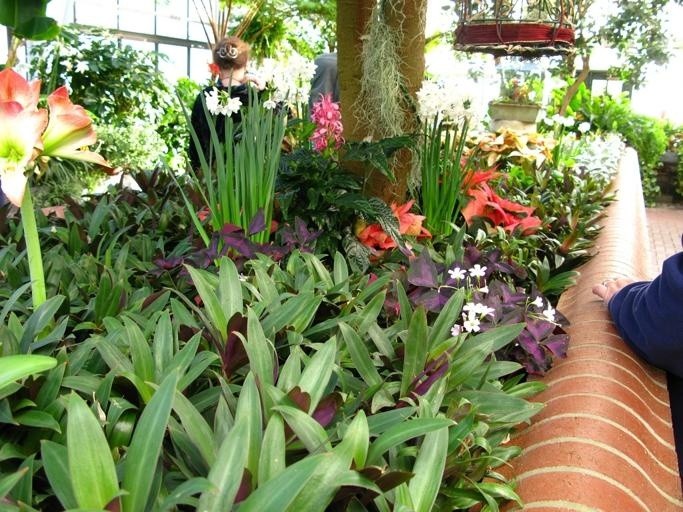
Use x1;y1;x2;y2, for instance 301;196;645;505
489;79;540;123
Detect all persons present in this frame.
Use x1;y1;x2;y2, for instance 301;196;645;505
591;231;682;431
187;35;295;173
307;46;340;113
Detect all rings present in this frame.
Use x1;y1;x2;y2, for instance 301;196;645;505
599;280;610;286
